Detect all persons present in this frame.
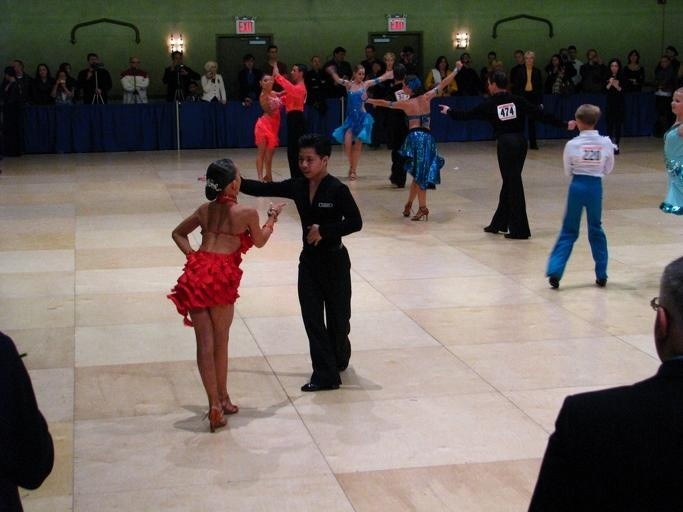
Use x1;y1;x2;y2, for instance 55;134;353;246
372;51;405;94
527;255;683;512
652;55;678;138
326;65;394;181
511;51;544;150
438;70;532;240
273;59;307;179
162;50;201;103
423;55;458;98
559;48;578;87
306;55;327;102
568;46;584;86
622;49;646;90
659;87;683;217
322;46;353;99
510;49;524;85
185;81;202;103
0;66;23;105
454;52;482;97
664;45;680;77
50;68;75;104
59;62;81;104
601;58;630;155
12;59;31;104
0;329;56;512
254;61;287;184
372;63;410;188
237;53;262;104
394;46;417;66
166;158;287;433
197;131;363;392
31;63;56;104
480;51;496;76
200;61;227;105
360;44;385;81
543;103;615;289
262;45;289;92
119;56;150;105
78;53;113;106
360;60;464;222
543;54;570;96
578;49;602;86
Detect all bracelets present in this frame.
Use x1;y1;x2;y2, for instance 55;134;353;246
261;223;275;235
267;207;280;220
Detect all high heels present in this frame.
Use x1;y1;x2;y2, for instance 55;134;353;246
413;205;430;221
222;398;239;413
264;175;271;181
349;169;357;181
404;204;412;216
209;408;226;432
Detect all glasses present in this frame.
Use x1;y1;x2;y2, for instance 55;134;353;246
650;297;664;310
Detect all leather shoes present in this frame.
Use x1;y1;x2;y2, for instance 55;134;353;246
596;277;606;286
485;226;506;234
301;381;339;390
549;275;560;289
505;233;529;241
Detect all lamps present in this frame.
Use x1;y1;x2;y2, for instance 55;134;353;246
165;31;187;56
452;31;470;50
